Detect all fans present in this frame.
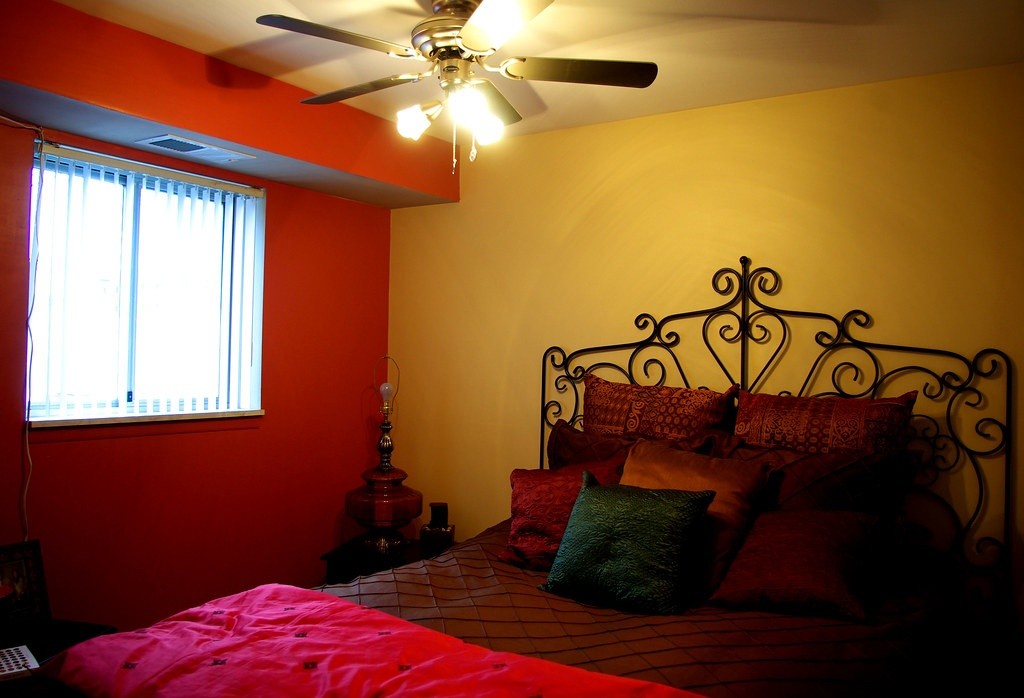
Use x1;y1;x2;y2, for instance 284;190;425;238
256;0;658;127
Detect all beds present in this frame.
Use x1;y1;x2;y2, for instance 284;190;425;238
56;256;1017;698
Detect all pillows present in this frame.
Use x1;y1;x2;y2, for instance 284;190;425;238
733;386;918;464
583;371;740;457
546;420;634;489
708;512;868;621
620;437;770;593
498;466;616;570
709;434;866;514
537;468;717;618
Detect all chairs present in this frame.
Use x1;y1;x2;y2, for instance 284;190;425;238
0;536;117;698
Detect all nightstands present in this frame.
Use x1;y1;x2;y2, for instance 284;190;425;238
320;532;460;584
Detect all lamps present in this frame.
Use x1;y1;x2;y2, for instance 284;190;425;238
344;355;422;551
396;58;505;173
